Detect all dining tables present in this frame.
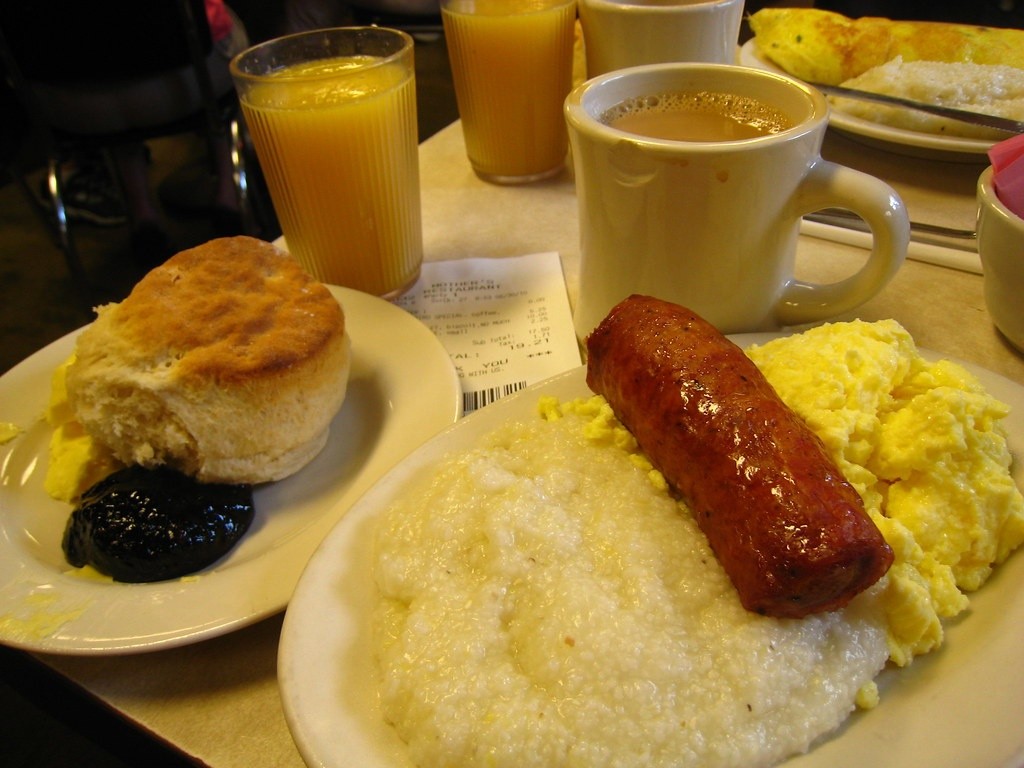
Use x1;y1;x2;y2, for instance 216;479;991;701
0;115;1023;767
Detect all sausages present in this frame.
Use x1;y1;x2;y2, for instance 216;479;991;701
584;292;894;615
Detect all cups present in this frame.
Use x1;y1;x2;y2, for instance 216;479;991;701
573;0;745;103
561;62;910;340
437;0;578;185
977;165;1024;351
230;23;425;305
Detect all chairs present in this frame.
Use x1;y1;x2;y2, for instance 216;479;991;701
0;0;267;290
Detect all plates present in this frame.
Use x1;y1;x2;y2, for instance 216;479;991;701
801;77;1024;136
740;33;1021;163
274;326;1022;768
1;282;464;657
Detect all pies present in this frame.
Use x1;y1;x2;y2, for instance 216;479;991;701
749;6;1024;86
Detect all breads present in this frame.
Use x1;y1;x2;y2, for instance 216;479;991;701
63;236;351;486
